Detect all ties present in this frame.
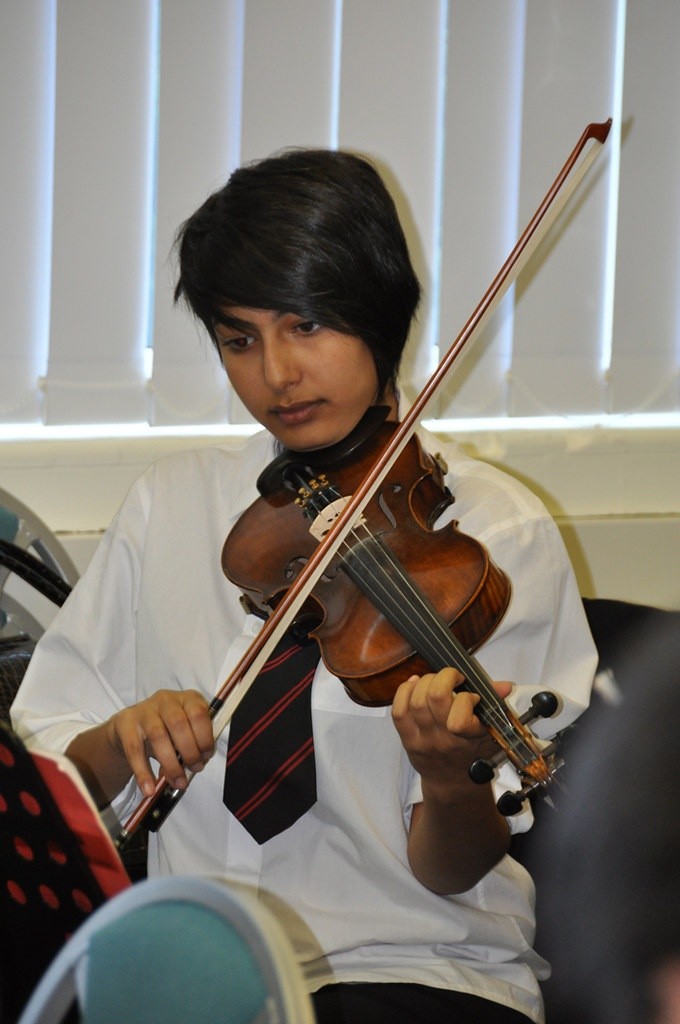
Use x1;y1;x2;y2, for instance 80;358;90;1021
222;628;322;847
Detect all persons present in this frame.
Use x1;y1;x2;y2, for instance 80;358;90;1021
10;151;598;1024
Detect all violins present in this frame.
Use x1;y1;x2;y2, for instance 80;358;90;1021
219;419;569;817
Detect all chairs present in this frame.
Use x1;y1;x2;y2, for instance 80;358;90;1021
20;876;315;1024
510;599;680;1024
